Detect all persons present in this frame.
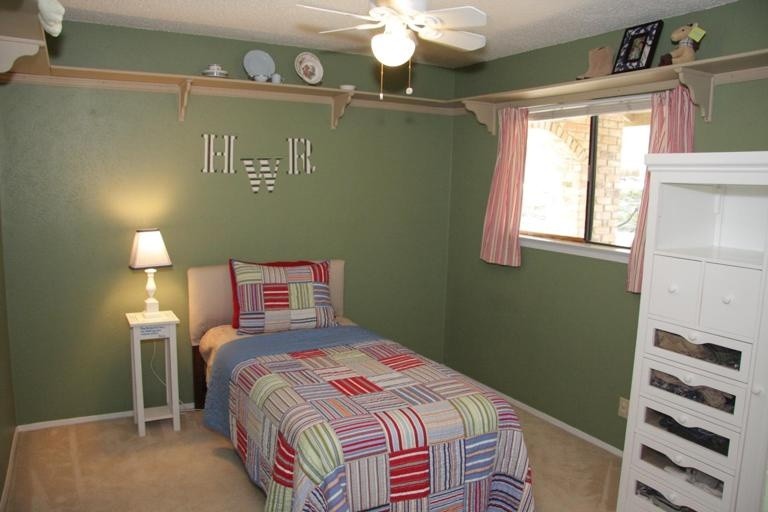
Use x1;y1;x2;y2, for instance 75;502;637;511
632;41;644;59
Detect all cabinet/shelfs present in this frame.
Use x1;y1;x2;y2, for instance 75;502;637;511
617;152;768;512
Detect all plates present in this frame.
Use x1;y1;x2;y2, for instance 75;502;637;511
294;52;324;84
242;49;275;78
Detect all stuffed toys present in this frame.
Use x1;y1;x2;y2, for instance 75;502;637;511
660;25;695;66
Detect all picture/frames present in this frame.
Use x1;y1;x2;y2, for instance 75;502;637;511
611;20;663;74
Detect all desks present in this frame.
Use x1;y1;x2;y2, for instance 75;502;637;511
125;311;181;437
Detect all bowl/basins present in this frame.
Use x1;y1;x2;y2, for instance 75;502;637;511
251;74;267;81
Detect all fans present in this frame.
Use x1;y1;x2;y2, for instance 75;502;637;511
297;0;486;52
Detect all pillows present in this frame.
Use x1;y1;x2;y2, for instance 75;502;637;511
234;261;337;333
229;261;317;328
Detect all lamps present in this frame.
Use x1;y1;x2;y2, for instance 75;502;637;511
128;228;172;321
370;25;416;67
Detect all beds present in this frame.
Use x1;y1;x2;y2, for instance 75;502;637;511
187;259;534;512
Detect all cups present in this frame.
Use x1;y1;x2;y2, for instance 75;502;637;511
271;73;282;82
